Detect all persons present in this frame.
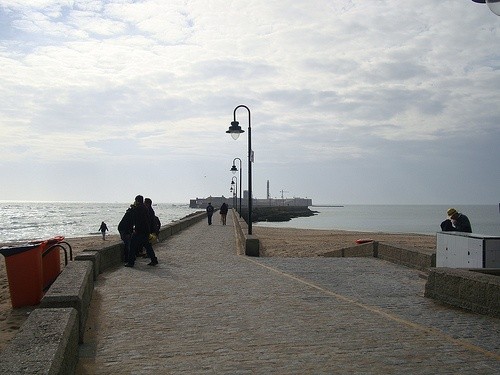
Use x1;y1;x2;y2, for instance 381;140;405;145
220;202;228;225
136;197;155;259
447;208;473;233
99;222;109;241
117;202;136;260
207;203;214;225
125;195;159;267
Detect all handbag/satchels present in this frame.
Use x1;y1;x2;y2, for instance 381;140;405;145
220;209;223;214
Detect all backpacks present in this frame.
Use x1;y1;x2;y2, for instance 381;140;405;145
150;215;161;235
208;207;212;213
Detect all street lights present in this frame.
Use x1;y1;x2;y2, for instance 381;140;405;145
230;176;237;211
226;105;254;234
229;158;242;218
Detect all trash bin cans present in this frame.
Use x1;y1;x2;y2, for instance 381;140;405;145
0;237;64;307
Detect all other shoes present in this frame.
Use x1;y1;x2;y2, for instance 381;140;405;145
147;261;158;265
124;263;134;267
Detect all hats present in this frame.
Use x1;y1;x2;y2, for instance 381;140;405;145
447;207;458;219
